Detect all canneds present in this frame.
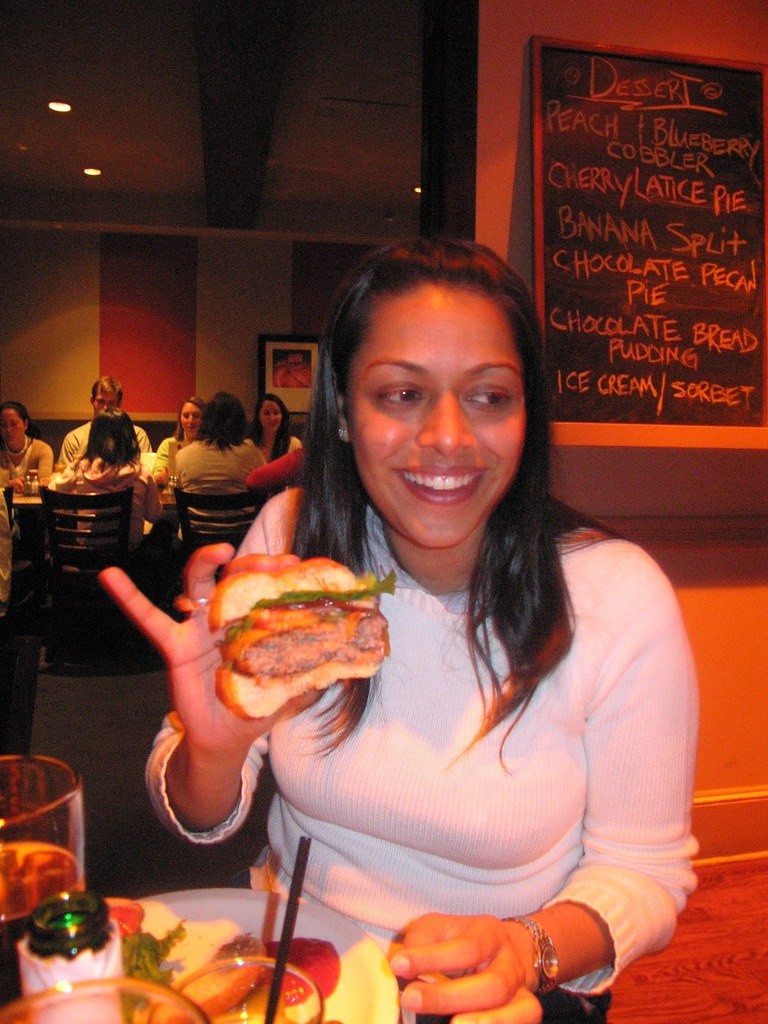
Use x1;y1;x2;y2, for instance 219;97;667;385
23;476;40;495
167;476;179;495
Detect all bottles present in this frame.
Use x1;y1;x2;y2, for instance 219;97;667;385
24;469;39;496
169;476;177;502
17;891;124;1000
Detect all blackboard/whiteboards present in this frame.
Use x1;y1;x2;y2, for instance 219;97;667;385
532;34;768;451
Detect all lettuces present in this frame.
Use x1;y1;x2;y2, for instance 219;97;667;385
119;918;185;985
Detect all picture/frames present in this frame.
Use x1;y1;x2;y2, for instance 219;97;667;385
258;333;323;415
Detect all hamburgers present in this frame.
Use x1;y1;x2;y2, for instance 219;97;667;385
209;557;397;720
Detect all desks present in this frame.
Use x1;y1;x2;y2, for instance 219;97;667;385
9;492;179;517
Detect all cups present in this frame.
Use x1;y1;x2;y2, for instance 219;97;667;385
0;754;86;1004
0;976;209;1024
144;955;325;1024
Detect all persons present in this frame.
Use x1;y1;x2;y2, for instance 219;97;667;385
273;350;312;388
93;229;701;1024
53;375;153;475
173;388;269;551
0;488;12;617
0;401;53;496
153;396;205;487
54;406;163;554
246;394;304;463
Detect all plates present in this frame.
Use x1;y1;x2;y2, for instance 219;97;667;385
127;887;400;1024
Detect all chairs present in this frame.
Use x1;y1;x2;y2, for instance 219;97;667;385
0;484;268;756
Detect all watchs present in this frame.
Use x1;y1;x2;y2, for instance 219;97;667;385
501;915;559;995
